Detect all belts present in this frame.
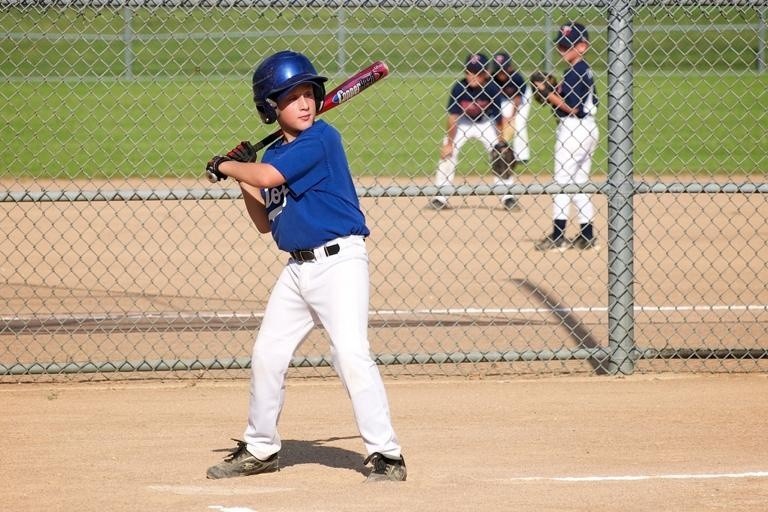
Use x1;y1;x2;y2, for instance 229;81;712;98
289;237;365;264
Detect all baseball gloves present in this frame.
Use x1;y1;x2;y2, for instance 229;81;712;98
531;71;559;106
492;143;516;179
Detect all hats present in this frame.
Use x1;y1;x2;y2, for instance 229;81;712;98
463;52;490;76
553;22;590;50
490;50;511;73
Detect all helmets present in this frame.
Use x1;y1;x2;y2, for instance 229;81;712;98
251;50;328;126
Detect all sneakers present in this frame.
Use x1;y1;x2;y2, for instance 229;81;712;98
503;196;522;213
534;233;564;252
564;235;598;250
204;443;281;480
360;452;409;486
427;199;452;212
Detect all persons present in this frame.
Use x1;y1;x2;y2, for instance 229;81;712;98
206;51;406;483
538;22;601;250
486;49;535;163
432;54;519;210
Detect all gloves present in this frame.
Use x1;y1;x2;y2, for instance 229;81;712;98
225;140;258;183
205;155;232;184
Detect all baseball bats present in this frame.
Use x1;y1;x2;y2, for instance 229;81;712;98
206;61;390;182
